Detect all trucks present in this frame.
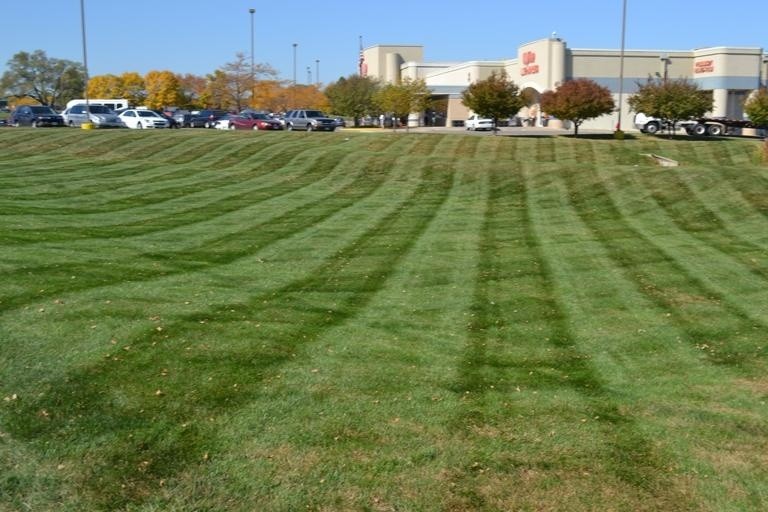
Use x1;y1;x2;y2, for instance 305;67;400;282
635;109;767;137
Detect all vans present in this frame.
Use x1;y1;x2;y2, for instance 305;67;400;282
62;99;128;112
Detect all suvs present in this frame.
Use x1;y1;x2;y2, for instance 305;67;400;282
281;109;338;131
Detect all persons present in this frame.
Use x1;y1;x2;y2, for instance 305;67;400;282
431;110;437;126
378;113;385;128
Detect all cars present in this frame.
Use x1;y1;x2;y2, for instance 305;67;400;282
118;109;170;130
359;109;443;128
62;102;126;130
8;104;65;128
146;106;287;131
332;117;347;128
464;114;494;131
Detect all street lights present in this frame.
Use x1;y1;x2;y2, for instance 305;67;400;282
247;7;256;107
306;58;321;87
659;54;674;139
291;43;298;87
